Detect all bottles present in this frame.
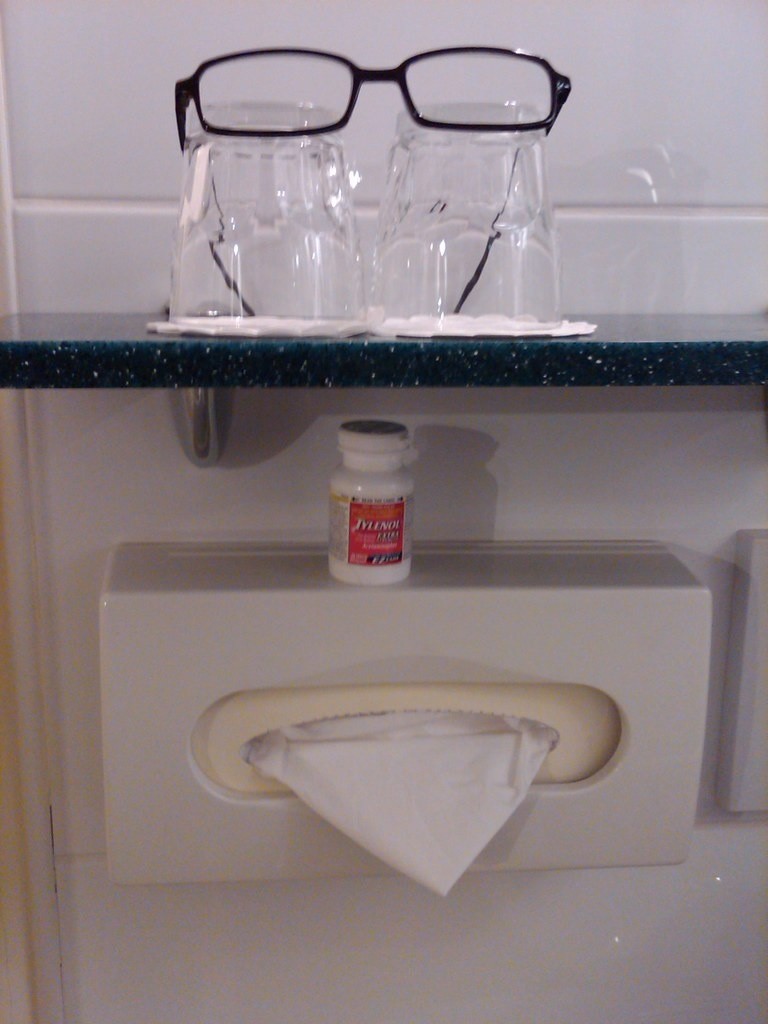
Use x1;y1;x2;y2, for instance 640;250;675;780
330;417;418;587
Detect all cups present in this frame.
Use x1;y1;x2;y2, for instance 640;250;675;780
170;98;363;326
374;107;556;323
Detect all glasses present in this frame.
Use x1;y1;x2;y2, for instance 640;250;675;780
173;45;573;315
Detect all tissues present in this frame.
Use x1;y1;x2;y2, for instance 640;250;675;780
96;546;713;896
